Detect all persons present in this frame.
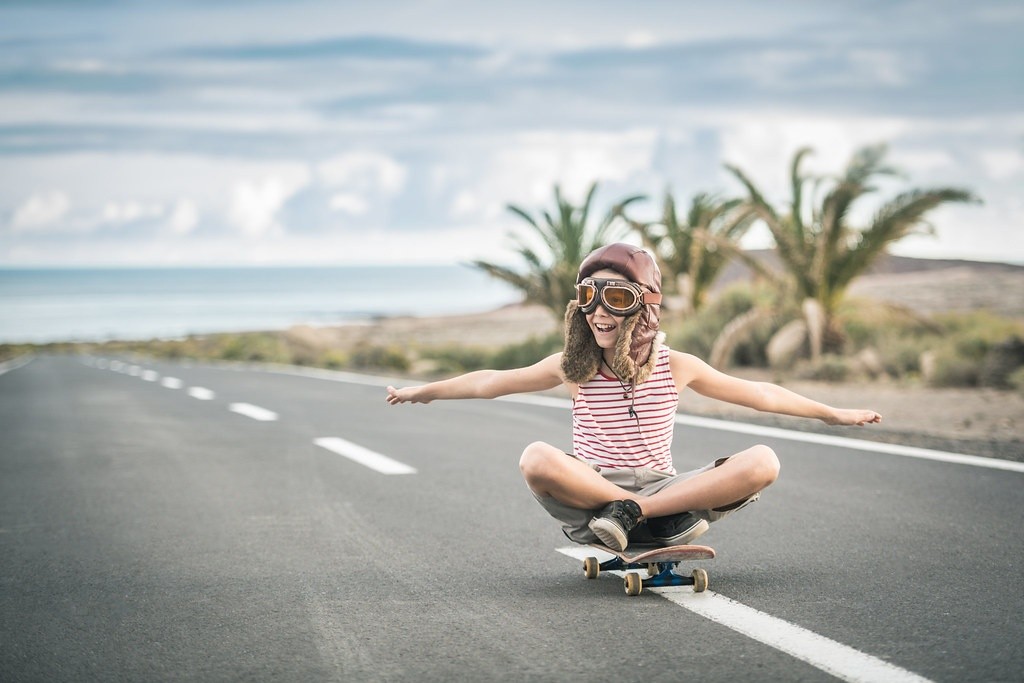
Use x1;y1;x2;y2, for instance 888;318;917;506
384;243;882;550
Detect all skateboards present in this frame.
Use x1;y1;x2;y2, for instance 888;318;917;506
562;526;716;598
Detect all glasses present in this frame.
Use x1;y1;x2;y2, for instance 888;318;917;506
575;276;662;317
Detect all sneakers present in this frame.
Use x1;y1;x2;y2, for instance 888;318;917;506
588;498;642;552
648;510;710;545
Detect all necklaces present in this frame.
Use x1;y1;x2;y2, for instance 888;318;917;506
601;355;635;399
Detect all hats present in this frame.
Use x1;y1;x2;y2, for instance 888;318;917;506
560;243;663;418
